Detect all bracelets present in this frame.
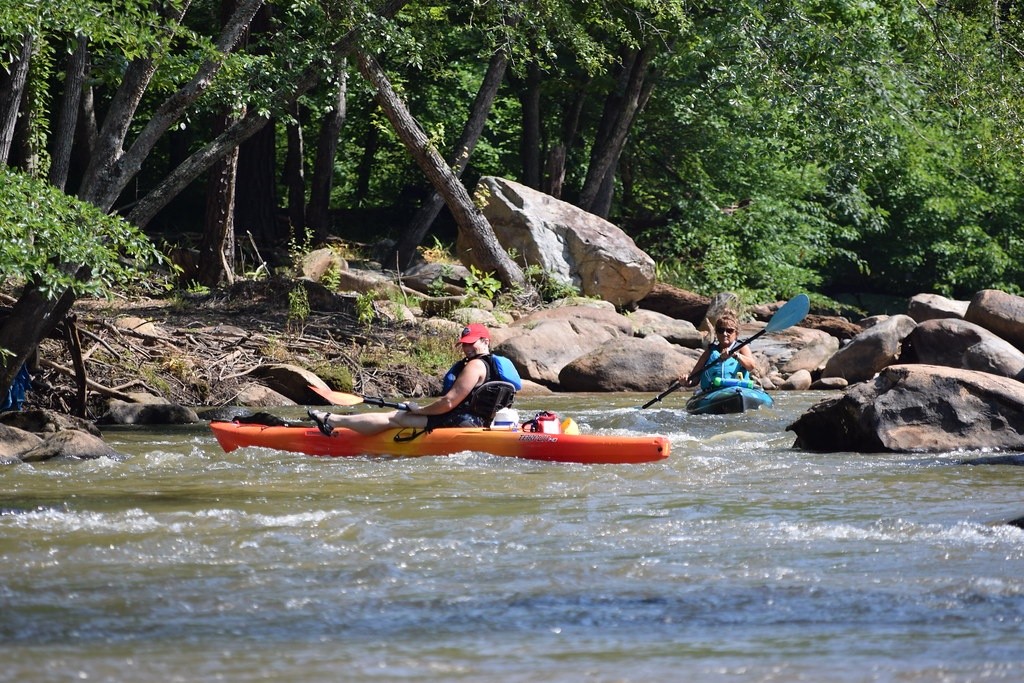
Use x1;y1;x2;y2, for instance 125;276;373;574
733;355;738;360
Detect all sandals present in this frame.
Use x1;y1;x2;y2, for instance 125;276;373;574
308;409;333;436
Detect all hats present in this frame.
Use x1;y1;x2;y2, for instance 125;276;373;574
455;324;490;347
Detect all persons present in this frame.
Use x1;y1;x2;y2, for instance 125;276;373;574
678;316;755;390
307;323;498;437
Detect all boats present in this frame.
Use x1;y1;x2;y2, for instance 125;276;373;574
685;386;772;414
212;419;669;464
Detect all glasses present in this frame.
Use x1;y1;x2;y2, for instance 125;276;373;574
718;328;734;334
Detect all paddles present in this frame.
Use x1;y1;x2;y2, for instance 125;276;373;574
307;385;411;412
642;293;811;409
